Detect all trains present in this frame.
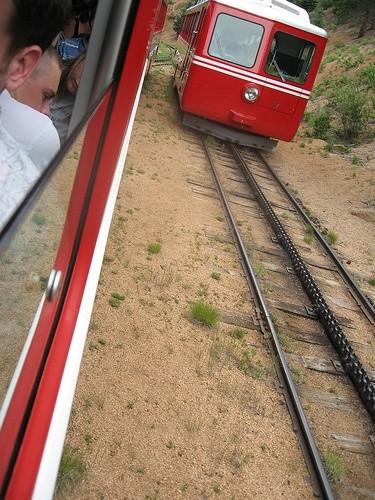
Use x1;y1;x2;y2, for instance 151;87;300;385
171;0;329;153
0;0;170;500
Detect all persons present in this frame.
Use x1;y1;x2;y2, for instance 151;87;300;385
1;0;97;234
